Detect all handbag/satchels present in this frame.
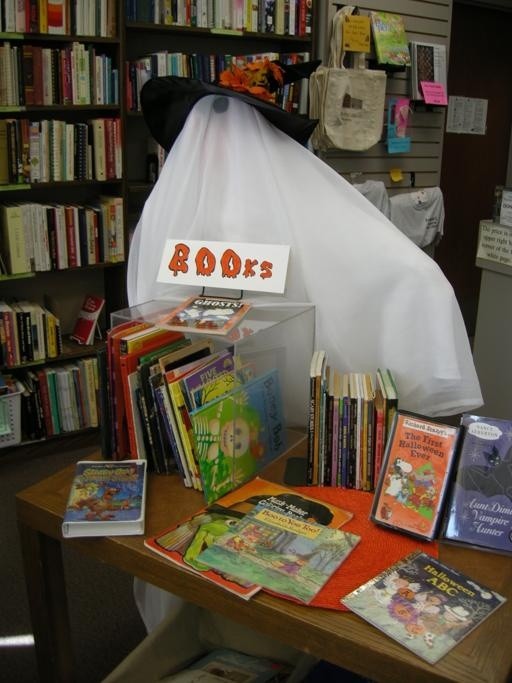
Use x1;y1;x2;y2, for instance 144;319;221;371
308;4;389;151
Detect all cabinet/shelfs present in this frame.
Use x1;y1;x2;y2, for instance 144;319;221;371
1;0;323;456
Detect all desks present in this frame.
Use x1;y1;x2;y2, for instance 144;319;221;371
11;431;512;677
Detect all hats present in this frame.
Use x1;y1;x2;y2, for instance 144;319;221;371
140;57;321;146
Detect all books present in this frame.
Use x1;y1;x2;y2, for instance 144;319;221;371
368;11;412;67
339;548;507;667
309;350;512;552
195;503;361;605
1;1;126;276
162;647;284;683
62;459;147;537
108;296;288;502
129;1;312;180
144;477;353;602
2;295;104;436
